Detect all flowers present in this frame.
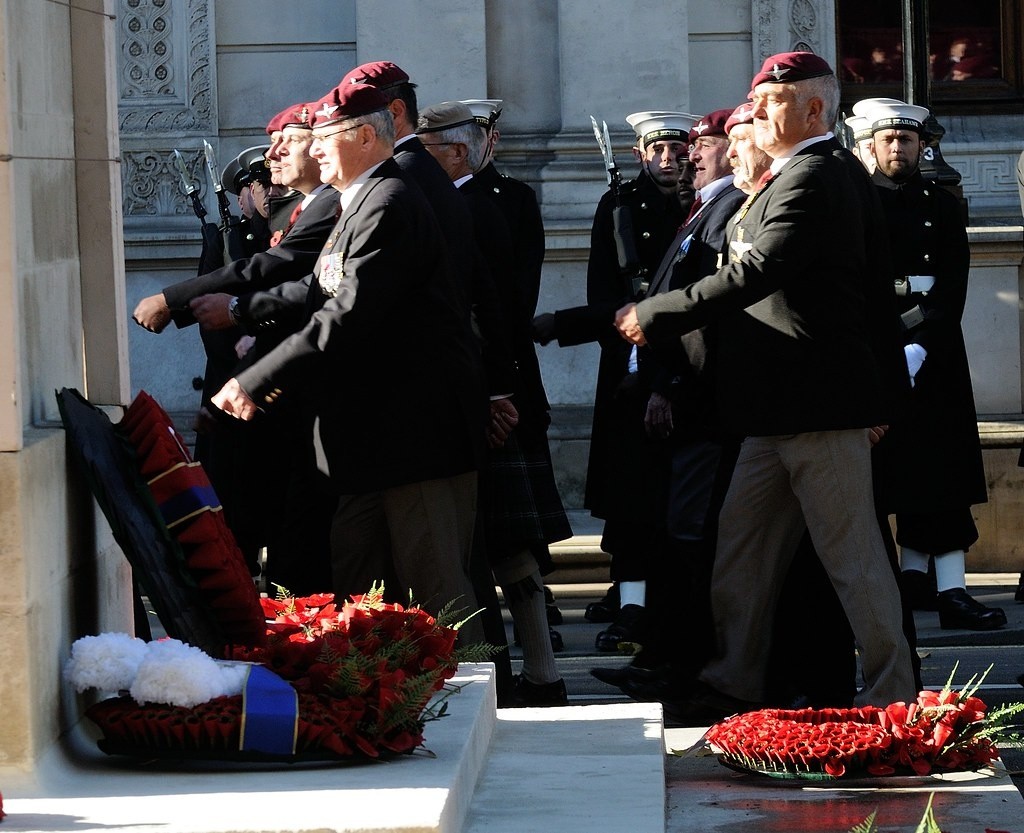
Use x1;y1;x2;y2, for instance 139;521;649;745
51;382;518;767
704;657;1024;782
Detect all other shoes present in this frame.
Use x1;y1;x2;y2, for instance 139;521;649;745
904;568;938;610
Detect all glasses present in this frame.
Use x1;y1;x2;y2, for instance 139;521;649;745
310;124;365;146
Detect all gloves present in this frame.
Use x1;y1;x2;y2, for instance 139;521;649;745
905;343;928;388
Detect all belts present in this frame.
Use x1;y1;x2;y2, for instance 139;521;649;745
896;276;935;295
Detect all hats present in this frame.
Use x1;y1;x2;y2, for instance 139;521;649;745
308;83;387;129
266;109;287;135
457;99;503;128
278;102;317;131
237;145;271;178
340;61;409;91
416;100;474;134
221;157;253;195
623;48;929;157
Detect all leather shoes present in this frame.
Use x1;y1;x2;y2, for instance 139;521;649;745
936;591;1008;632
500;575;838;727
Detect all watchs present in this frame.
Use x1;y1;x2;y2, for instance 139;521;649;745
228;293;242;321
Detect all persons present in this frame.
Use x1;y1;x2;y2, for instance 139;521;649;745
132;60;573;702
534;52;1024;725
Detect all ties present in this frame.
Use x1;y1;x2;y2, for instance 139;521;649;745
755;170;774;194
335;204;341;224
284;204;302;234
685;197;702;224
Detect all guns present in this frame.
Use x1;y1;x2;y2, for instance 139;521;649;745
590;114;624;190
172;147;220;247
601;118;650;298
202;136;241;231
842;111;851;152
